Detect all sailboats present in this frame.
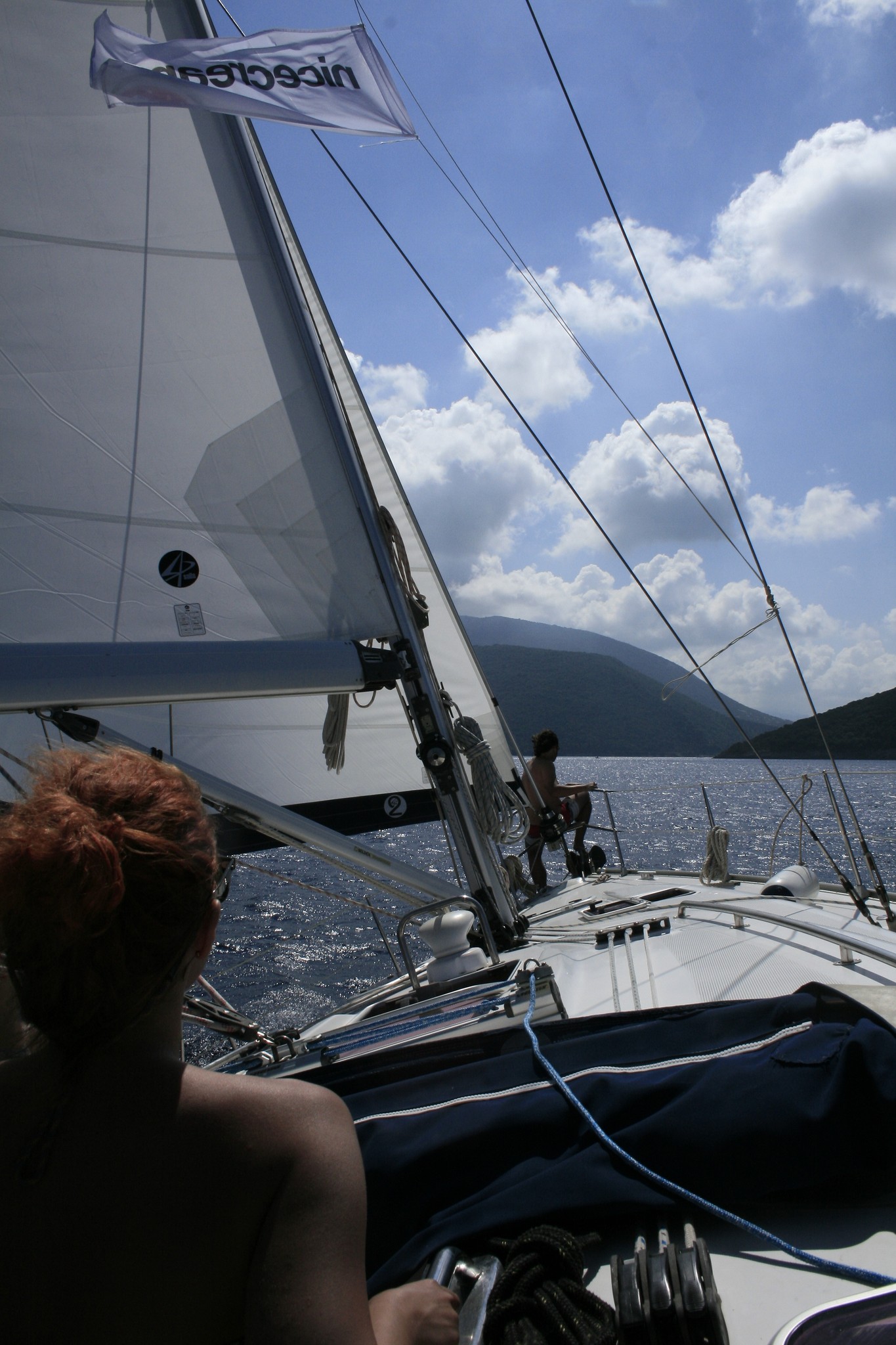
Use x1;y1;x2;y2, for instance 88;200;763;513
0;1;896;1345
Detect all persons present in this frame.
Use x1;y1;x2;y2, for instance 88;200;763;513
521;729;599;893
0;748;462;1345
